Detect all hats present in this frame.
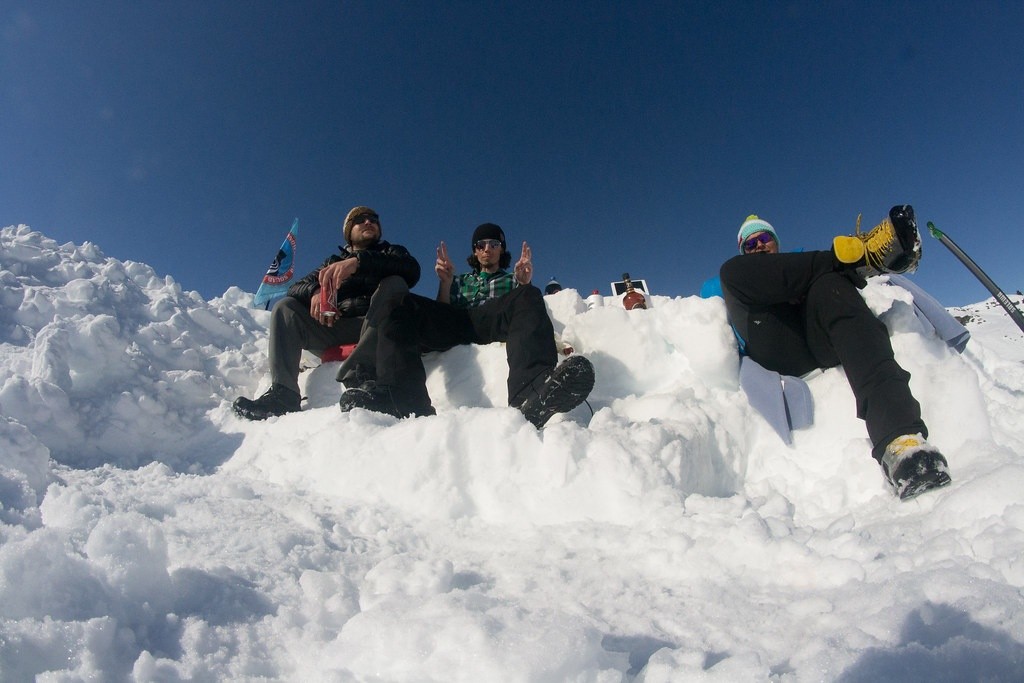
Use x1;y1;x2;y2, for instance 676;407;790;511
471;222;506;251
737;214;782;254
342;205;380;245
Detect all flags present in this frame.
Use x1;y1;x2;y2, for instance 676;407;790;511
254;217;300;307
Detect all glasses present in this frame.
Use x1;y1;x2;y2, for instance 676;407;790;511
474;239;501;249
353;212;377;224
742;232;771;251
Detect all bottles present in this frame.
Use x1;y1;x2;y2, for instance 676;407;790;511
622;273;647;310
320;274;336;317
587;290;604;310
543;276;562;295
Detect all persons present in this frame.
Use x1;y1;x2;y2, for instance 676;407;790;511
720;204;951;501
232;206;594;429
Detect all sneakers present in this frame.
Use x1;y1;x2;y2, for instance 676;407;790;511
340;380;374;411
880;432;952;499
832;203;923;277
233;383;302;420
380;401;435;417
520;356;595;430
